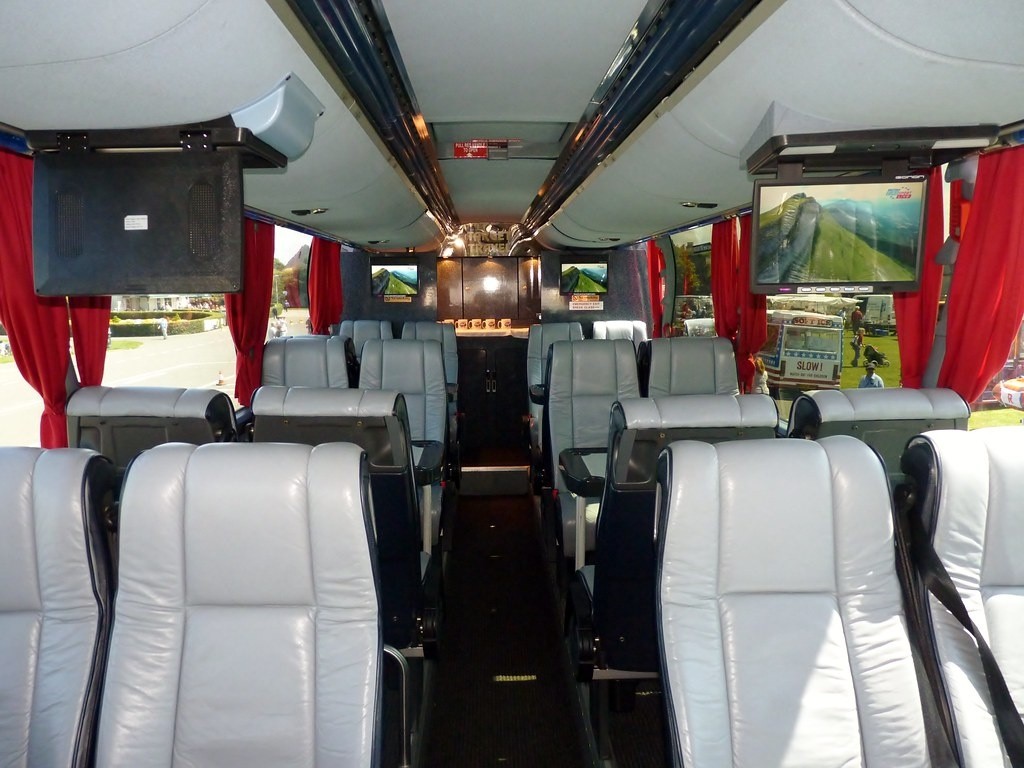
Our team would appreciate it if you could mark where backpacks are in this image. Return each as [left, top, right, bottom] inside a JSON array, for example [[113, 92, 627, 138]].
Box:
[[849, 334, 859, 349]]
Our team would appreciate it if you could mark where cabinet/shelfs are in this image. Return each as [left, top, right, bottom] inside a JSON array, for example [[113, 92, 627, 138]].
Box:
[[455, 336, 530, 470]]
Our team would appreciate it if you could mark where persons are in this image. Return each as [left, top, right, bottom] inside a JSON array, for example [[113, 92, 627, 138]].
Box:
[[157, 314, 168, 340], [284, 299, 289, 311], [269, 308, 286, 338], [837, 306, 879, 366], [858, 364, 884, 389]]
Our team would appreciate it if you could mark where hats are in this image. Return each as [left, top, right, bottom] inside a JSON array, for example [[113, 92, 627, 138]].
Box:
[[855, 306, 860, 309], [866, 363, 875, 370]]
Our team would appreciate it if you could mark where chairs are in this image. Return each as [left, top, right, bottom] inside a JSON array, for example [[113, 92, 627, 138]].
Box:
[[60, 384, 253, 597], [339, 321, 393, 364], [0, 446, 112, 768], [787, 386, 973, 513], [96, 441, 412, 768], [563, 393, 783, 768], [591, 320, 650, 364], [525, 321, 586, 453], [895, 429, 1024, 768], [637, 337, 740, 395], [356, 338, 453, 608], [648, 429, 955, 768], [250, 385, 443, 714], [261, 334, 357, 390], [528, 337, 645, 584], [400, 320, 460, 462]]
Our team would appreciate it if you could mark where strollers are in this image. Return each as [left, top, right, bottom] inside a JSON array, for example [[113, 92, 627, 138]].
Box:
[[862, 344, 891, 368]]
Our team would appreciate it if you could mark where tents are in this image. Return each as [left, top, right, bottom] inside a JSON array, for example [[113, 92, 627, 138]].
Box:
[[766, 294, 864, 312]]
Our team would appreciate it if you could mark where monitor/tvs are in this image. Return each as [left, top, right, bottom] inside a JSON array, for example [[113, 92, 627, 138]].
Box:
[[29, 152, 242, 296], [751, 178, 927, 293], [369, 257, 420, 297], [559, 261, 609, 295]]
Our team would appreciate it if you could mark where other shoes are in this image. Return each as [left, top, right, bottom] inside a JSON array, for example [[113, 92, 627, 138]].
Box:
[[851, 360, 858, 367]]
